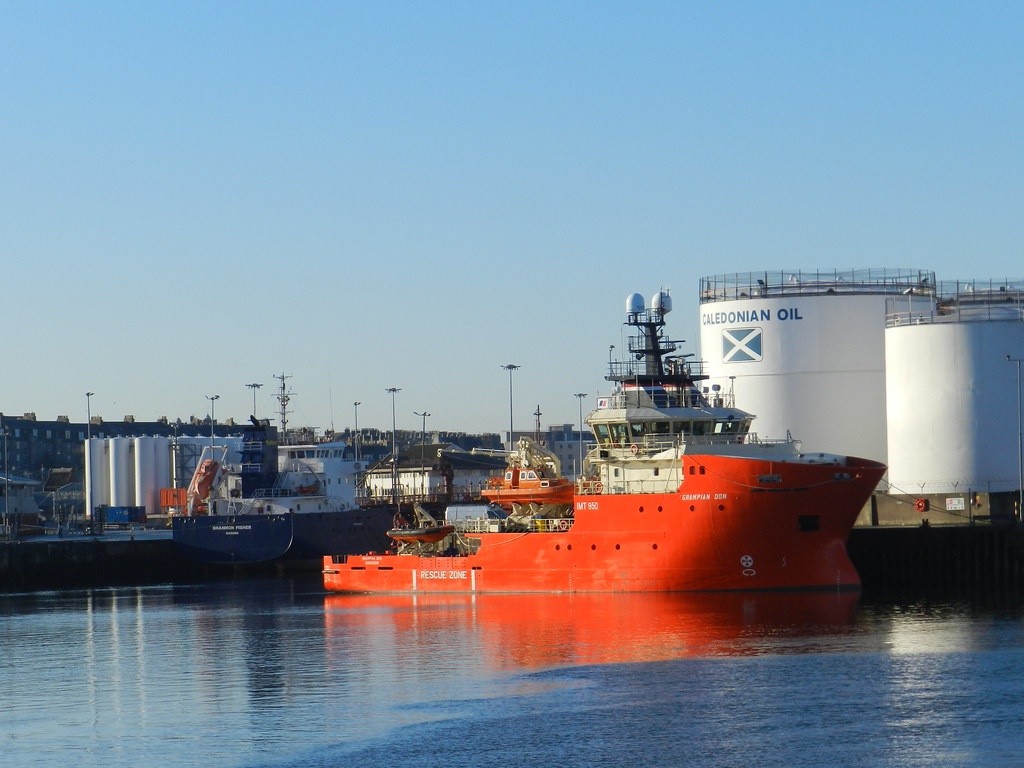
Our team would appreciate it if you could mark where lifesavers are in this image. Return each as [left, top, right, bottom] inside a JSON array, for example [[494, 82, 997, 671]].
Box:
[[630, 444, 638, 455], [914, 499, 926, 510], [595, 483, 603, 493], [559, 521, 568, 531]]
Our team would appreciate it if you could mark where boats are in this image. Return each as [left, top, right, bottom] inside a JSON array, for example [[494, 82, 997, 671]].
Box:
[[480, 474, 574, 508], [387, 519, 454, 543]]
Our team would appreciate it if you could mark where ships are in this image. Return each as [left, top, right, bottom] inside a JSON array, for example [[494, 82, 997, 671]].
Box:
[[319, 286, 888, 595]]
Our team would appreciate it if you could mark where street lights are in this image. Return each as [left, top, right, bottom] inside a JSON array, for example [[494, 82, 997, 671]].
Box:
[[573, 393, 588, 477], [245, 383, 263, 418], [383, 388, 402, 499], [353, 401, 362, 498], [413, 412, 431, 508], [500, 364, 521, 452], [205, 395, 220, 461], [86, 391, 95, 524], [0, 432, 12, 531]]
